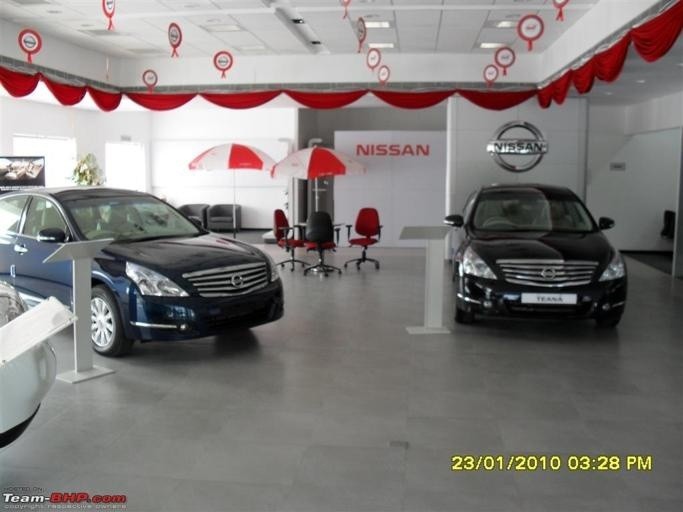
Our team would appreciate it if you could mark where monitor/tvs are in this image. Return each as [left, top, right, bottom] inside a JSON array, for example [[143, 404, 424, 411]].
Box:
[[0, 155, 46, 195]]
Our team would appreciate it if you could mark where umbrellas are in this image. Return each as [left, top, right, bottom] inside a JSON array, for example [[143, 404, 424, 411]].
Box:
[[271, 144, 367, 213], [189, 141, 278, 239]]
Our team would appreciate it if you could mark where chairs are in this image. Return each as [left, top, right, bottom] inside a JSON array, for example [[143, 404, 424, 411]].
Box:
[[274, 208, 380, 277], [176, 204, 241, 239]]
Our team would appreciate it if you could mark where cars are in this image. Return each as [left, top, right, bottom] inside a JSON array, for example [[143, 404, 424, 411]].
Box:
[[444, 183, 628, 327]]
